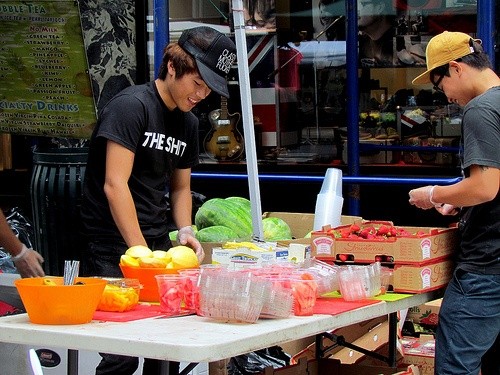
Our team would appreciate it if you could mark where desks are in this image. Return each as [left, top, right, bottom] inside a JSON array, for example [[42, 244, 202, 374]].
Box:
[[0, 287, 387, 375], [314, 286, 452, 375], [341, 138, 396, 163]]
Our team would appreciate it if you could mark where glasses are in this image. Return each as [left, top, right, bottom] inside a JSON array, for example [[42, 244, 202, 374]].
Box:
[[433, 61, 461, 92]]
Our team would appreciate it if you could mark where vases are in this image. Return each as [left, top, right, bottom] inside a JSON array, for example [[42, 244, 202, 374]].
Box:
[[370, 122, 391, 138]]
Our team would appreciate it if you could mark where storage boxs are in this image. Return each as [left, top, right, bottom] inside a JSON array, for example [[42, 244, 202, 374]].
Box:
[[197, 211, 458, 375]]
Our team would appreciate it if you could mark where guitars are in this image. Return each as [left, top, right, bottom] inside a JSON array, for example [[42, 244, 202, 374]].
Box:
[[203, 96, 244, 161]]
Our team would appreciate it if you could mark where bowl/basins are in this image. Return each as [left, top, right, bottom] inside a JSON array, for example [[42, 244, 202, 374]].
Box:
[[92, 277, 144, 312], [14, 277, 108, 325], [118, 263, 199, 302], [196, 258, 392, 323]]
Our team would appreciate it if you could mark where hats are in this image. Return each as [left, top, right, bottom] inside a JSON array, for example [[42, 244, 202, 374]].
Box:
[[413, 31, 482, 83], [178, 27, 236, 98]]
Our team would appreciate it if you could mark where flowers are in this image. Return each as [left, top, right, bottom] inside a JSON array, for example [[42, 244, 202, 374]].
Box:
[[360, 111, 395, 128]]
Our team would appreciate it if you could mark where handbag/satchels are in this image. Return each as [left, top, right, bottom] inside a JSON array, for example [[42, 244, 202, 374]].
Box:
[[225, 345, 291, 375]]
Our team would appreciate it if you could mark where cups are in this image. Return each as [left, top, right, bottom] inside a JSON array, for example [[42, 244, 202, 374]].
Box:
[[313, 167, 344, 231], [178, 269, 200, 309], [155, 274, 186, 314]]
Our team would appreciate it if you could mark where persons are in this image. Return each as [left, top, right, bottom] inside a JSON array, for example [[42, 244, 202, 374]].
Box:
[[0, 209, 45, 278], [318, 0, 346, 41], [408, 30, 500, 375], [77, 26, 237, 375], [357, 0, 396, 56]]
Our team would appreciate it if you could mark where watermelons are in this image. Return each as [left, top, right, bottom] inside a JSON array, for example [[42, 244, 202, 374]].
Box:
[[194, 196, 292, 242], [158, 266, 318, 315]]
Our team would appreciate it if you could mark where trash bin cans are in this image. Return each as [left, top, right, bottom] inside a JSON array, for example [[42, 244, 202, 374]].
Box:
[[30, 144, 90, 277]]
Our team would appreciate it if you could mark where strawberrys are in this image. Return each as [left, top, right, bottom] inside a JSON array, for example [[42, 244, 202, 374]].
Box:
[[327, 224, 426, 262]]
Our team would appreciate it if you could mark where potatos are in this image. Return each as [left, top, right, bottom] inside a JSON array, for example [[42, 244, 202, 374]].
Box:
[[120, 244, 199, 269]]
